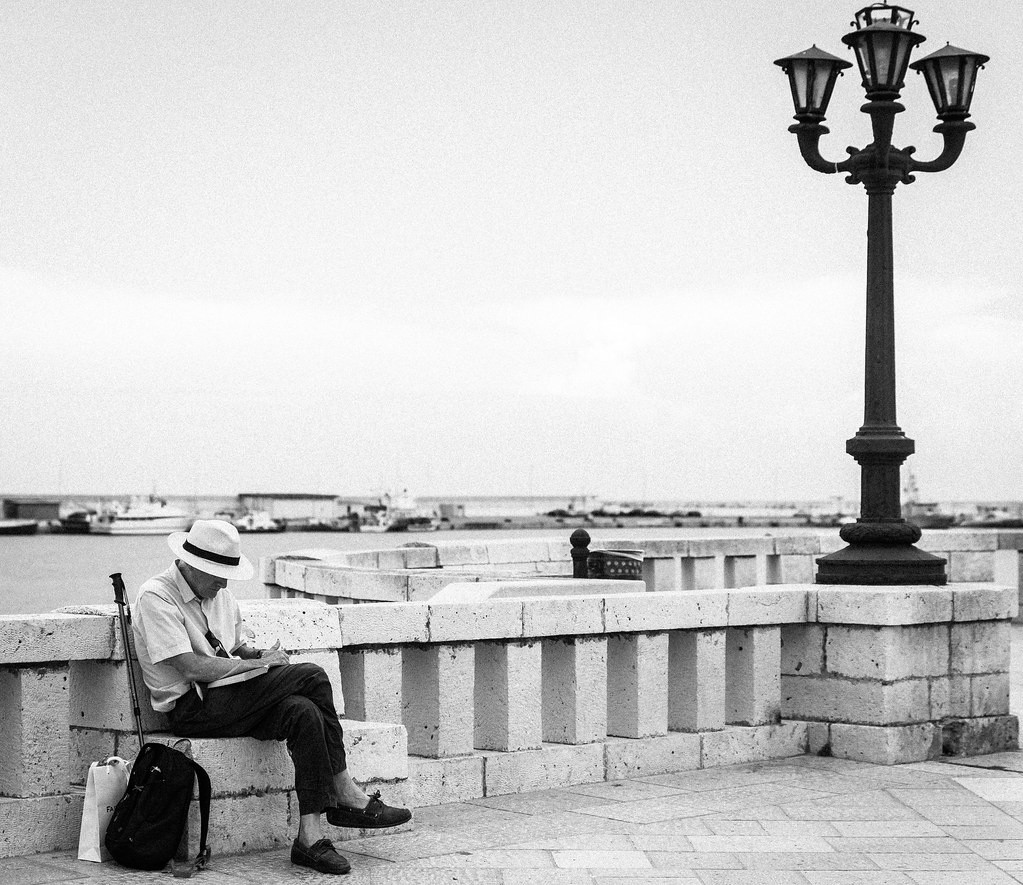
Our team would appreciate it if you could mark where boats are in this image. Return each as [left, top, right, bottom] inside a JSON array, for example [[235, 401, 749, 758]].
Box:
[[320, 493, 438, 533], [0, 515, 40, 537], [233, 508, 287, 533], [46, 520, 64, 536], [56, 504, 188, 535]]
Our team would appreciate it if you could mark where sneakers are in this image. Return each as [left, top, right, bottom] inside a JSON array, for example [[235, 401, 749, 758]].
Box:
[[290, 836, 351, 874], [326, 790, 412, 828]]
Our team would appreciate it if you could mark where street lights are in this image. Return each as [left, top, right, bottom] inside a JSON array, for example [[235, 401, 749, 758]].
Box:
[[769, 0, 989, 583]]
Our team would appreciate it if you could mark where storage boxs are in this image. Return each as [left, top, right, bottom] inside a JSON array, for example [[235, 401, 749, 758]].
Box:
[[587, 554, 645, 580]]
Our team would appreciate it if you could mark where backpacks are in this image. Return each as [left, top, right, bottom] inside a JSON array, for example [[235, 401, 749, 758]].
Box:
[[105, 738, 211, 878]]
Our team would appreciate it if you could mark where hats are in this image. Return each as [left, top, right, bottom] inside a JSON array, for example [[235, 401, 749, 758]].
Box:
[[168, 520, 255, 580]]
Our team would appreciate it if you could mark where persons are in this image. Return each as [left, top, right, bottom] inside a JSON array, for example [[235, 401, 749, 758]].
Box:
[[133, 520, 411, 875]]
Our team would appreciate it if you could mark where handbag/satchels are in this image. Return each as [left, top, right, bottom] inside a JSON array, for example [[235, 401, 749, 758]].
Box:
[[78, 756, 131, 863]]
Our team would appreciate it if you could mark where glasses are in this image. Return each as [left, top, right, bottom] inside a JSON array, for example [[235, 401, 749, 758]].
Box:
[[205, 630, 230, 659]]
